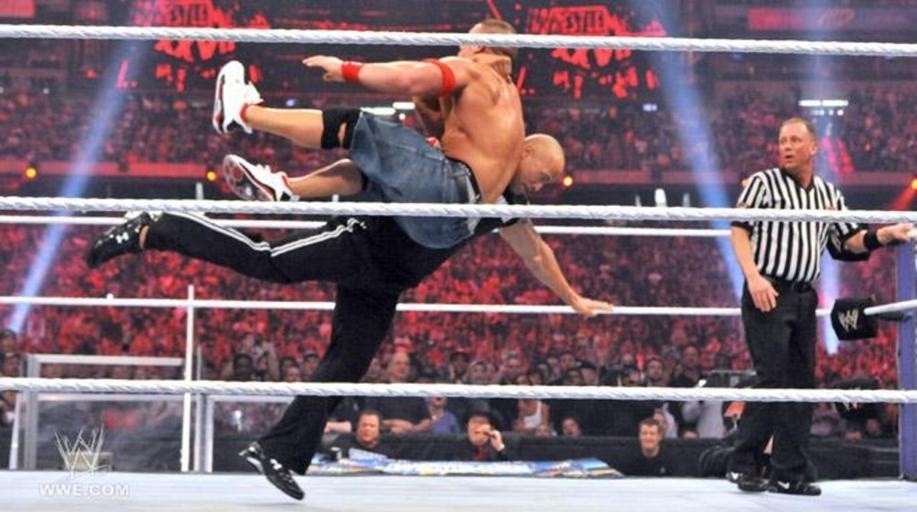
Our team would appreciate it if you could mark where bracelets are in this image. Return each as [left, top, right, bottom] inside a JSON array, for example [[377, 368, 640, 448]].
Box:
[[340, 60, 365, 87]]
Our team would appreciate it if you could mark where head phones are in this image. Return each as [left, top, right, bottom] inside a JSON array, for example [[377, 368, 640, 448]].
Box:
[[352, 409, 385, 433]]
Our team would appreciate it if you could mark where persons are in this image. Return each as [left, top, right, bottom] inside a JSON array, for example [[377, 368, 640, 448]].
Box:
[[732, 117, 915, 497], [212, 18, 529, 248], [77, 132, 615, 501], [0, 75, 916, 477]]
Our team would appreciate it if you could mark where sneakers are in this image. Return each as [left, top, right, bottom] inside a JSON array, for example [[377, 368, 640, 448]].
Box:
[[768, 477, 821, 496], [85, 210, 155, 270], [223, 153, 301, 202], [238, 442, 304, 500], [212, 59, 264, 135], [726, 470, 768, 491]]
